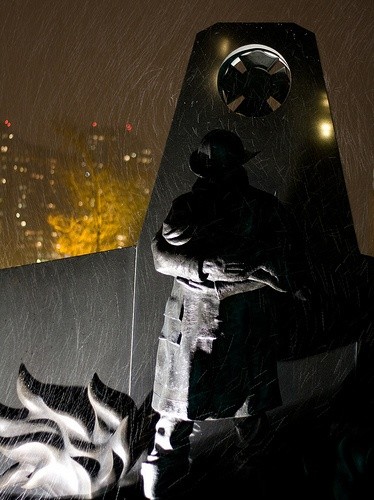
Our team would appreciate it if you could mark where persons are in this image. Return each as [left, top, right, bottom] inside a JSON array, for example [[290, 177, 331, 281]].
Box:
[[137, 128, 312, 500]]
